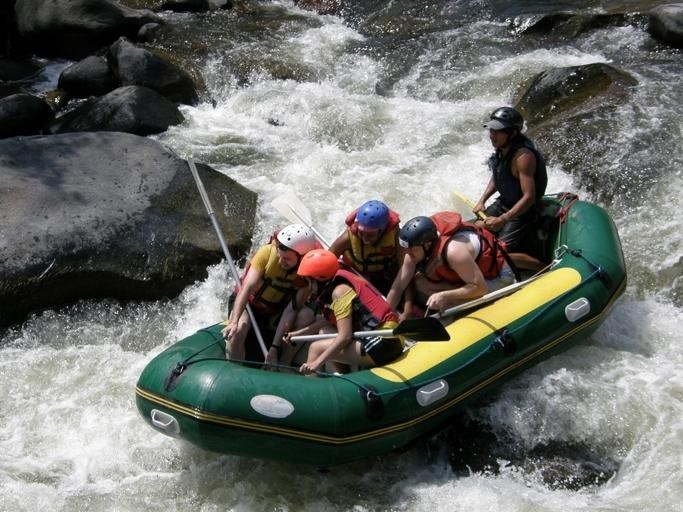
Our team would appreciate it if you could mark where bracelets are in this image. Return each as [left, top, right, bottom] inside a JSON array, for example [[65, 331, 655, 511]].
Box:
[[502, 213, 510, 222], [270, 345, 281, 351]]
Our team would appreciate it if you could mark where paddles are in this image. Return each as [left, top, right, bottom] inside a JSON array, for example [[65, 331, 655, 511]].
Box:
[[283, 318, 451, 348], [271, 190, 400, 314], [429, 274, 543, 320], [187, 156, 269, 362]]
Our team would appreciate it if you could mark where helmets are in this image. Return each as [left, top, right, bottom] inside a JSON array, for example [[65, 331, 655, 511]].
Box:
[[490, 107, 524, 132], [296, 249, 339, 281], [276, 224, 316, 255], [356, 200, 389, 236], [398, 215, 437, 249]]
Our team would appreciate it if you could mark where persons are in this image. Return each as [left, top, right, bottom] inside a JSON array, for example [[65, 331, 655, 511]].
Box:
[[472, 106, 547, 273], [220, 222, 315, 373], [281, 248, 406, 376], [385, 212, 516, 312], [328, 200, 414, 326]]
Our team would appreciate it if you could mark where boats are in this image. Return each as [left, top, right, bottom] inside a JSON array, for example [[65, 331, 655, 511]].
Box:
[[134, 192, 627, 467]]
